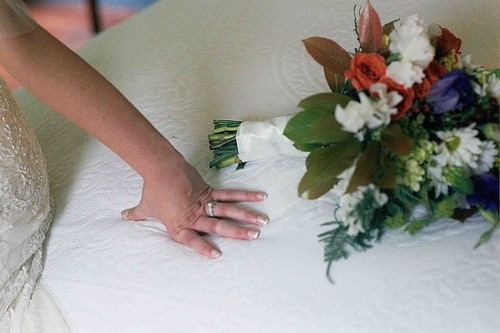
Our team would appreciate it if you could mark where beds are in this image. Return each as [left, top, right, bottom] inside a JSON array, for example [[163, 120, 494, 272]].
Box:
[[9, 0, 498, 333]]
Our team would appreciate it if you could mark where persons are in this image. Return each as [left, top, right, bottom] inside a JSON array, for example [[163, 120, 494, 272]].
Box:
[[0, 0, 270, 323]]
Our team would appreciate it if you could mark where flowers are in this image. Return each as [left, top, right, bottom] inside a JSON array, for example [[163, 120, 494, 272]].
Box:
[[208, 0, 499, 283]]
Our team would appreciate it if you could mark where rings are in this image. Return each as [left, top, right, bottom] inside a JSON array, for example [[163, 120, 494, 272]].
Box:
[[207, 200, 218, 218]]
